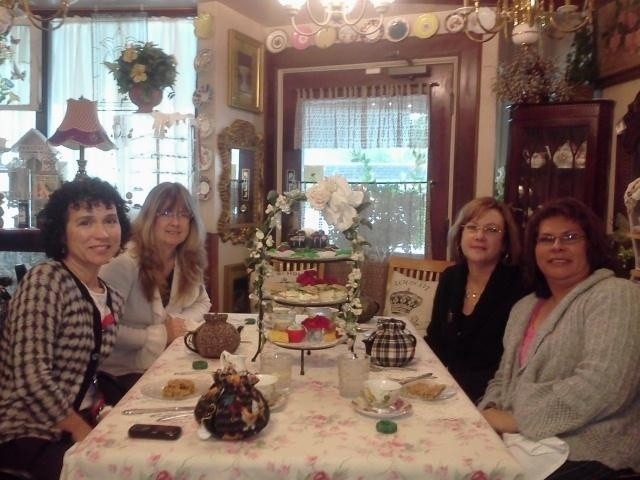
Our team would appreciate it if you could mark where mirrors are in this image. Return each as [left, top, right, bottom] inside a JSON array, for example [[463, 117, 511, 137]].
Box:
[[217, 119, 268, 245]]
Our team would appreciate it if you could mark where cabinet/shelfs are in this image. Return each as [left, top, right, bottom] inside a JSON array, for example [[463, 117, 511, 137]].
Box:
[[503, 99, 615, 234]]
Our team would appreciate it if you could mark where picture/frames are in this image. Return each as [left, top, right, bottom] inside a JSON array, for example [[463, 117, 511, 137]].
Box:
[[223, 262, 250, 313], [240, 168, 250, 201], [589, 0, 640, 89], [285, 169, 295, 193], [0, 15, 39, 111], [227, 29, 266, 113]]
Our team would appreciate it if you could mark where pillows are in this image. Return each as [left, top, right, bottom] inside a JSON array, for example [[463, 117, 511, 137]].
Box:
[[383, 271, 439, 337]]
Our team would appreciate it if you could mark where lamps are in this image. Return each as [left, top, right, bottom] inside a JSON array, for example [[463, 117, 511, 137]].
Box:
[[0, 0, 78, 31], [47, 96, 118, 179]]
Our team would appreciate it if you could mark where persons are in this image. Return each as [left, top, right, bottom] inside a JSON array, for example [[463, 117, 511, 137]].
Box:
[[1, 175, 129, 479], [474, 198, 639, 479], [421, 196, 538, 404], [97, 181, 211, 402]]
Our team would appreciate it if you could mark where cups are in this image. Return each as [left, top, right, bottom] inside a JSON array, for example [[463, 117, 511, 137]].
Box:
[[261, 353, 291, 392], [247, 374, 278, 405], [362, 381, 403, 408], [335, 352, 370, 398]]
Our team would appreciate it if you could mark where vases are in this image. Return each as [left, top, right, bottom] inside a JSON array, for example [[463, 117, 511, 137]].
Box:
[[129, 84, 162, 114]]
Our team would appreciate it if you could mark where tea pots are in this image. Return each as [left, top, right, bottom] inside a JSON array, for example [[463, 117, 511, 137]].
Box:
[[184, 312, 244, 358], [193, 364, 271, 441], [361, 318, 416, 367], [522, 140, 588, 170]]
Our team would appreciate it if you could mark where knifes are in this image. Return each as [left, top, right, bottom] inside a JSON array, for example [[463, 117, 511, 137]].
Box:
[[123, 406, 203, 415], [398, 372, 433, 385]]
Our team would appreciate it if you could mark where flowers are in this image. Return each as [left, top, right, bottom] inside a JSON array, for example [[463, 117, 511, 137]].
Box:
[[248, 172, 372, 349], [93, 34, 180, 99]]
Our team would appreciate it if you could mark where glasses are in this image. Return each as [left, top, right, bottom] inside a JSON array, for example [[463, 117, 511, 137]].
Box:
[[461, 223, 501, 235], [537, 231, 585, 244], [158, 209, 194, 220]]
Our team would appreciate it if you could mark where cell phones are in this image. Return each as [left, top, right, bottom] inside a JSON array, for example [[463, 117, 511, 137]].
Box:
[[128, 424, 181, 440]]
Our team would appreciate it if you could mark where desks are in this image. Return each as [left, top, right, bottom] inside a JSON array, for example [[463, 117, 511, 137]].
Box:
[[0, 227, 47, 252]]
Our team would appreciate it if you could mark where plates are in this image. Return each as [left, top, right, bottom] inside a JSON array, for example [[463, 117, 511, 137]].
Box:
[[413, 13, 439, 39], [265, 28, 288, 54], [192, 175, 213, 201], [141, 382, 216, 401], [338, 24, 358, 45], [194, 48, 216, 74], [192, 147, 213, 171], [402, 387, 457, 401], [291, 27, 313, 50], [316, 27, 337, 49], [351, 395, 411, 417], [468, 6, 496, 34], [192, 84, 216, 112], [196, 113, 214, 138], [445, 12, 466, 34], [194, 26, 214, 39], [360, 20, 385, 44], [385, 18, 410, 43]]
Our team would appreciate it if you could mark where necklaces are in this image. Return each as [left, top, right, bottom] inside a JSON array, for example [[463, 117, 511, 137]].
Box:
[[468, 272, 484, 299]]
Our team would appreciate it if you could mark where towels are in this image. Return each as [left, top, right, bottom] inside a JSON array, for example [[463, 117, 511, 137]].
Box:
[[499, 430, 569, 480]]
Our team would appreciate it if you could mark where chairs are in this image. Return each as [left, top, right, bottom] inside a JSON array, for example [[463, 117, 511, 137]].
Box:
[[15, 264, 29, 282], [385, 256, 451, 300]]
